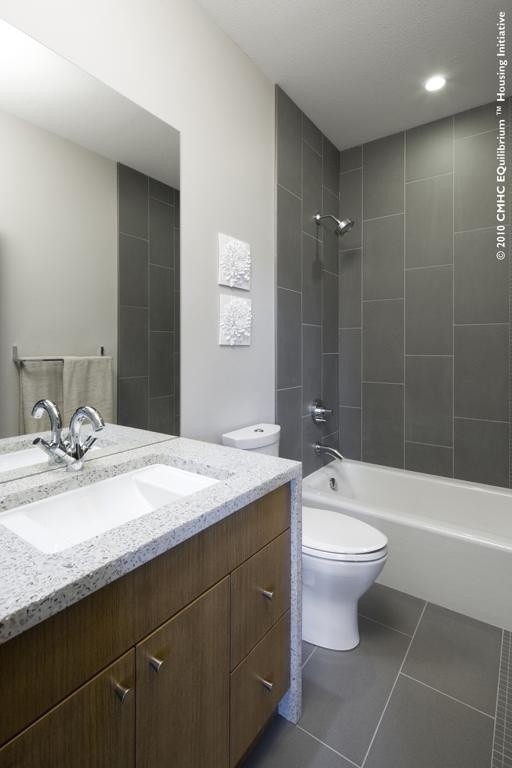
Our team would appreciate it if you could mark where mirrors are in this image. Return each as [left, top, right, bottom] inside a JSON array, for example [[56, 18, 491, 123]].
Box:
[[0, 18, 182, 488]]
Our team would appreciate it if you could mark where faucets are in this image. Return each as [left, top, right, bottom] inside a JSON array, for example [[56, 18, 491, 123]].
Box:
[[51, 406, 105, 472], [30, 396, 69, 461], [320, 444, 347, 464]]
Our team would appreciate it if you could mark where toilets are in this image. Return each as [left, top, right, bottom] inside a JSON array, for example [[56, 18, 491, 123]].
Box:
[[223, 423, 388, 651]]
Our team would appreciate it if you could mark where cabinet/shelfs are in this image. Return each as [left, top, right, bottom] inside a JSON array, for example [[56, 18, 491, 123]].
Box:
[[0, 478, 290, 768]]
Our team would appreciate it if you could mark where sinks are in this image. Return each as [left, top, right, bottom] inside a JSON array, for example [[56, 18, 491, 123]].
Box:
[[1, 455, 234, 555]]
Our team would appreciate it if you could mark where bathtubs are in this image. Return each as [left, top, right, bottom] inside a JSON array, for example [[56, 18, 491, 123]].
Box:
[[300, 460, 511, 635]]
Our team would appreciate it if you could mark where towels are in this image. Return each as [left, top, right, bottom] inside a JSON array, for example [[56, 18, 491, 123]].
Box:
[[17, 355, 113, 437]]
